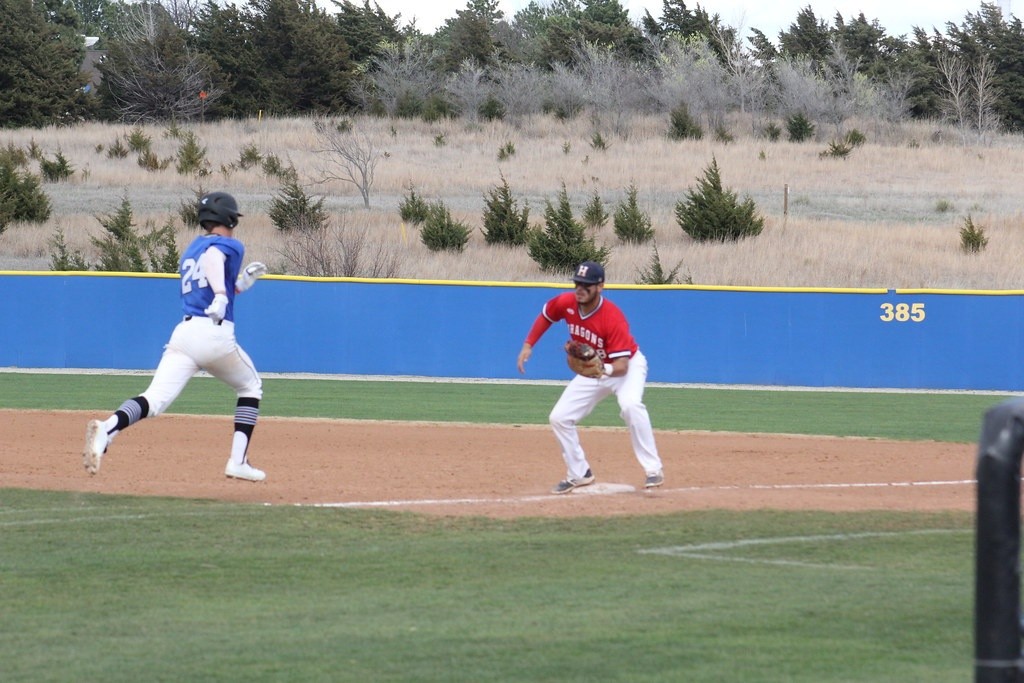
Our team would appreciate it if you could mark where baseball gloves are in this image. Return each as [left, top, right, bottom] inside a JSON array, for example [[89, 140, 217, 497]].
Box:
[[565, 339, 606, 379]]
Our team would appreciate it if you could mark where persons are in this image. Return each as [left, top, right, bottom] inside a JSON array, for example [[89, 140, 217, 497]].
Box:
[[517, 260, 665, 493], [82, 191, 266, 480]]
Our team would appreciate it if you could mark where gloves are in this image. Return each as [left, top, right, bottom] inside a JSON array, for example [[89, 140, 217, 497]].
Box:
[[235, 261, 268, 293], [204, 293, 230, 326]]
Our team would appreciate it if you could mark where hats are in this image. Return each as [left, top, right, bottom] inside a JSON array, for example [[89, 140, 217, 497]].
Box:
[[569, 262, 606, 285]]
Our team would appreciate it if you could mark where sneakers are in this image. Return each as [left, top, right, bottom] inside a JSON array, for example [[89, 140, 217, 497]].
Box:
[[81, 419, 110, 474], [224, 458, 266, 482], [645, 476, 663, 488], [549, 469, 596, 494]]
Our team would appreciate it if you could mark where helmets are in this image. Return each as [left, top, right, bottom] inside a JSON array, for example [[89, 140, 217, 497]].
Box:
[[197, 192, 243, 229]]
